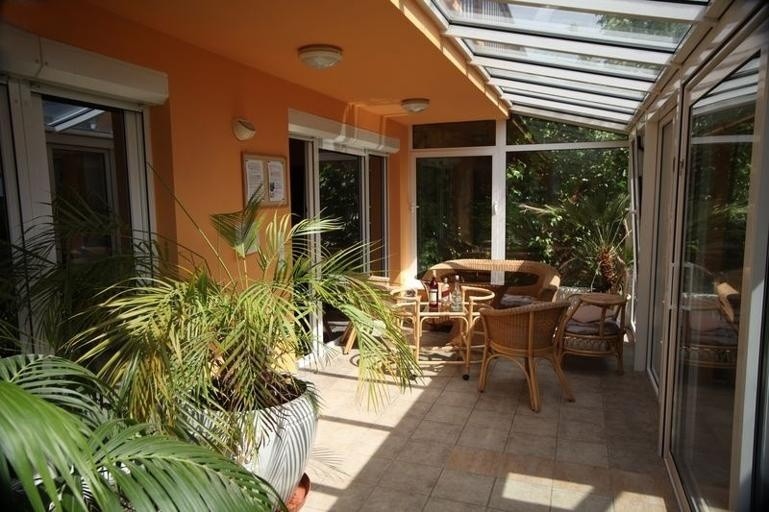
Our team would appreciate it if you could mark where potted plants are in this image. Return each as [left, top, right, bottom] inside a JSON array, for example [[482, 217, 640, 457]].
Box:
[[0, 177, 423, 511]]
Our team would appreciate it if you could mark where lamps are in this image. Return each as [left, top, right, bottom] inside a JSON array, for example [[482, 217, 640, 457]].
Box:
[[400, 98, 430, 113], [299, 44, 343, 70]]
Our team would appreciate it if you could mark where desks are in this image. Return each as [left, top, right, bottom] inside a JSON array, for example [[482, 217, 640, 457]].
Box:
[[557, 289, 627, 378]]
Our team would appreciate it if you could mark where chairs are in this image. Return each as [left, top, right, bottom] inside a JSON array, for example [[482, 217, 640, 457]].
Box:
[[338, 284, 576, 414]]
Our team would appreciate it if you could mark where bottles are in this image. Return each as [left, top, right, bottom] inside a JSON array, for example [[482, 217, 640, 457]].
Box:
[[429, 275, 463, 312]]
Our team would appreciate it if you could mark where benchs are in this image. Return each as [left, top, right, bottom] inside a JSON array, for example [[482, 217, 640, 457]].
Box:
[[422, 257, 561, 314]]
